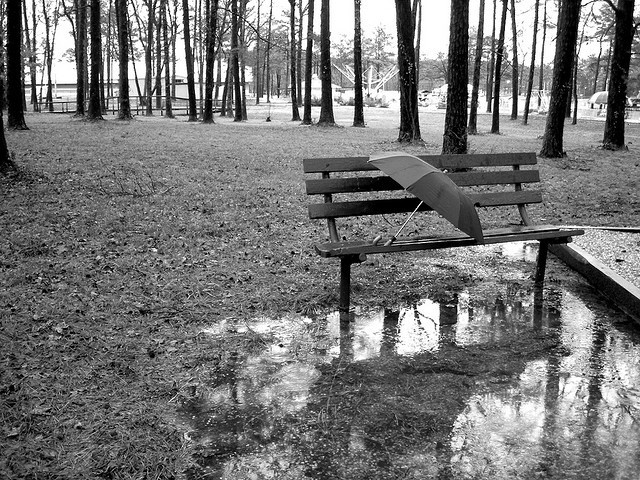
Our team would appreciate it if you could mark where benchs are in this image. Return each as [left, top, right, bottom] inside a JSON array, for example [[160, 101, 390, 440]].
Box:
[[303, 151, 585, 315]]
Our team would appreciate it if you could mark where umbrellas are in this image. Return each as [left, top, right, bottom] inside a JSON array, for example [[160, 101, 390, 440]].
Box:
[[367, 151, 487, 245], [587, 91, 633, 107]]
